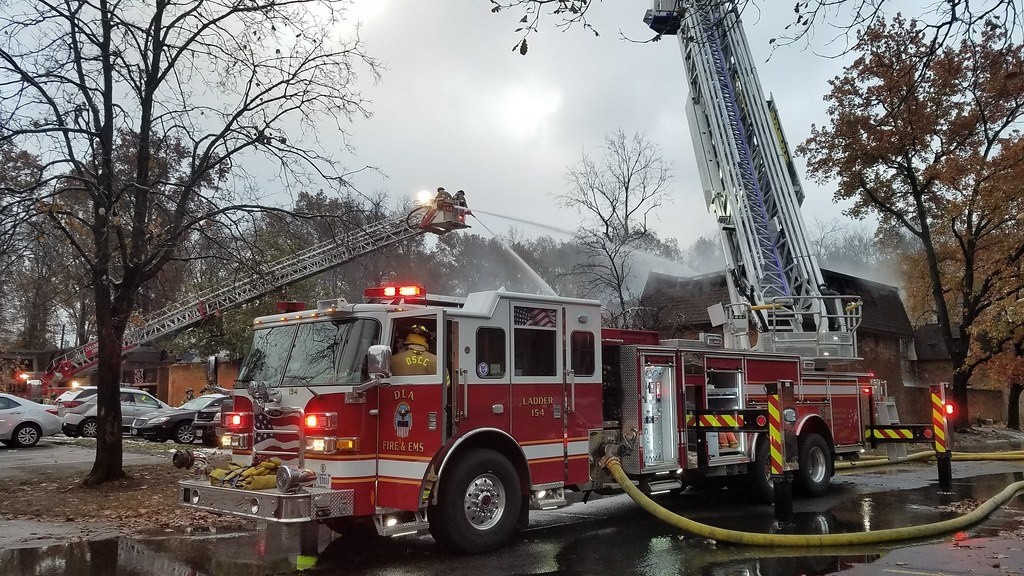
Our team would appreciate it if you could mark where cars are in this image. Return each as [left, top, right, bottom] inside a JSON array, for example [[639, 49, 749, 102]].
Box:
[[52, 385, 100, 417], [128, 392, 230, 445], [191, 396, 231, 445], [62, 388, 179, 438], [0, 392, 63, 449]]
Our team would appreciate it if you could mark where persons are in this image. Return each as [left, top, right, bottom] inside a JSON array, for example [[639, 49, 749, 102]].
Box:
[[141, 388, 151, 401], [47, 392, 58, 405], [181, 387, 197, 406], [390, 325, 450, 387], [431, 186, 452, 208], [453, 189, 468, 208], [160, 347, 177, 360], [392, 335, 406, 355]]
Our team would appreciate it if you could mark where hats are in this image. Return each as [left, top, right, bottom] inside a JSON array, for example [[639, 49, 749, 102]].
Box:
[[458, 190, 465, 195], [437, 187, 445, 192]]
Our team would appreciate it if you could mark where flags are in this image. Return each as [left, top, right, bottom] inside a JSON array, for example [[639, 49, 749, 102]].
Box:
[[514, 306, 556, 327]]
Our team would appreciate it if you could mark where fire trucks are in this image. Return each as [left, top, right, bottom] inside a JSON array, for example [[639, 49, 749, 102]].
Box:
[[170, 0, 958, 555], [8, 199, 471, 406]]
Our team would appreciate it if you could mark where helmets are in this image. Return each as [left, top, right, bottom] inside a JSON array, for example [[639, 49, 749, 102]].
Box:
[[402, 333, 429, 351]]
